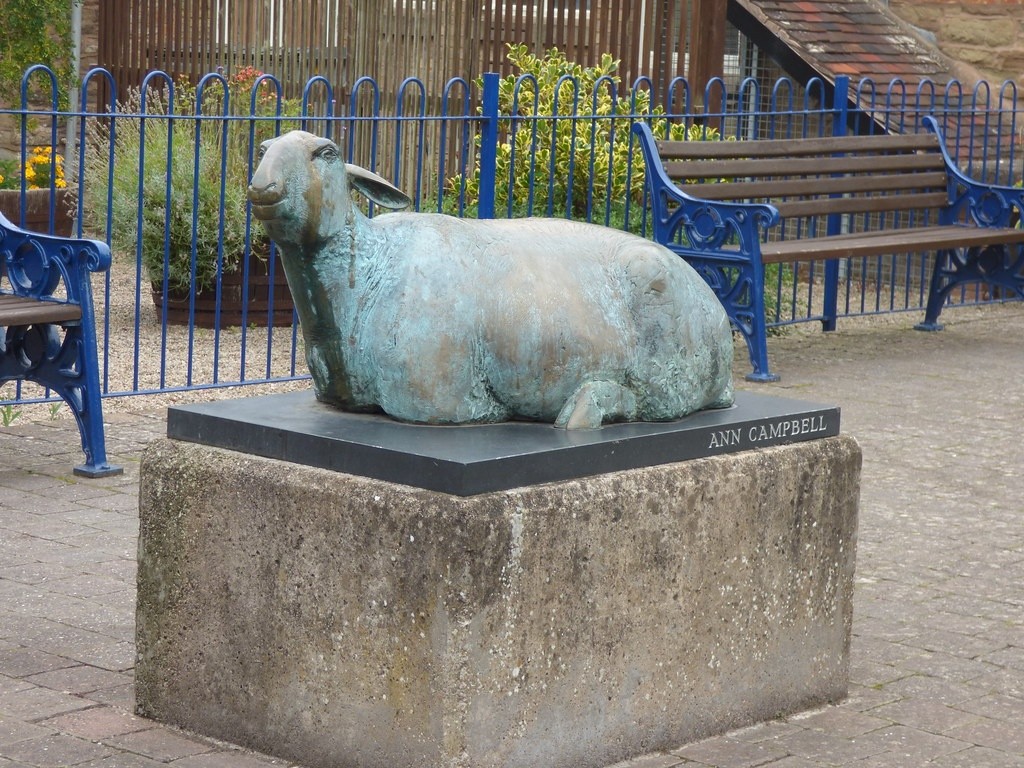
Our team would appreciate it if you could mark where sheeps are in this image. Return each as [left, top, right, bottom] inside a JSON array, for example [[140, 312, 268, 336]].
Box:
[[247, 130, 734, 431]]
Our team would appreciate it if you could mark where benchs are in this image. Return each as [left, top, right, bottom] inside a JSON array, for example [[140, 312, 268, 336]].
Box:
[[0, 211, 125, 480], [631, 112, 1024, 386]]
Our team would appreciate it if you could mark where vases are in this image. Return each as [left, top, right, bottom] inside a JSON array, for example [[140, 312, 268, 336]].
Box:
[[0, 181, 79, 276]]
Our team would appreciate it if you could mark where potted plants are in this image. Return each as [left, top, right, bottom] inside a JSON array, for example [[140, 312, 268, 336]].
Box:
[[70, 66, 313, 327]]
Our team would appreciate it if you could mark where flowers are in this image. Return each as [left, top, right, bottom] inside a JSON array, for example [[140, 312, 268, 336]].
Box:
[[0, 146, 68, 190]]
